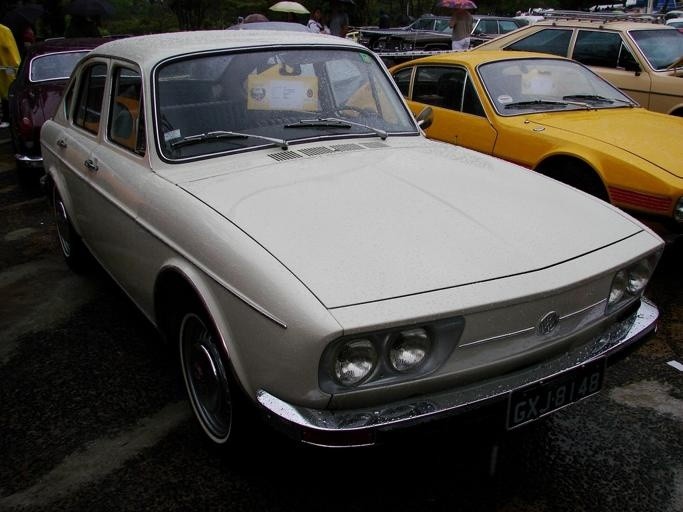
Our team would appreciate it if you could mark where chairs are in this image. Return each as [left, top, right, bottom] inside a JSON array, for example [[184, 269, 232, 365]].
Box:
[[92, 79, 312, 148], [393, 72, 543, 116]]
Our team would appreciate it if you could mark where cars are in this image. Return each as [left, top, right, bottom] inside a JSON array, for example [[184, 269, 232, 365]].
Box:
[[8, 36, 146, 171], [346, 5, 683, 67], [40, 28, 667, 460], [470, 18, 683, 118], [343, 49, 683, 223]]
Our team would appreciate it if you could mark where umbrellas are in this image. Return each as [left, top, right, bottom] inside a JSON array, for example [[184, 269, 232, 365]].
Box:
[[268, 0, 312, 22], [435, 0, 478, 19]]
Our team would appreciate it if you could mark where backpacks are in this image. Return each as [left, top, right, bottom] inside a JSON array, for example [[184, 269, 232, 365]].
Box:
[[321, 24, 331, 34]]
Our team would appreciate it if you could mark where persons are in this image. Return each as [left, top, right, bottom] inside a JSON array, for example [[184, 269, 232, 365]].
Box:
[[448, 8, 473, 84], [16, 26, 35, 61], [308, 7, 328, 35], [241, 7, 269, 23], [328, 1, 350, 38], [283, 13, 298, 23], [378, 8, 389, 45]]
